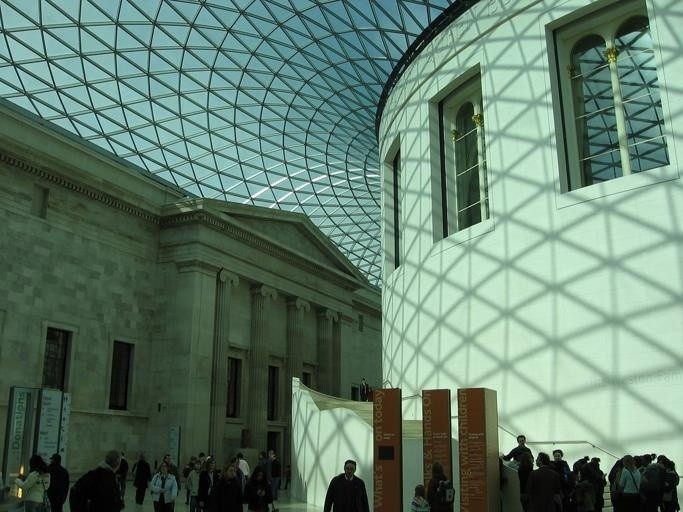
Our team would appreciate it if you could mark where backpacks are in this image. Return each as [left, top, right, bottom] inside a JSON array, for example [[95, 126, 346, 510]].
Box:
[[70, 472, 98, 511]]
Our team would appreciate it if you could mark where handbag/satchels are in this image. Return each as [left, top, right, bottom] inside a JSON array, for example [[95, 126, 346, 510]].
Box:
[[43, 489, 52, 510]]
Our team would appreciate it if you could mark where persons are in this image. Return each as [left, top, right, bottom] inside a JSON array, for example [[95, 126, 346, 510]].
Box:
[[410, 484, 430, 512], [499, 434, 680, 512], [15, 449, 281, 511], [323, 460, 369, 512], [425, 462, 455, 512], [360, 377, 369, 402]]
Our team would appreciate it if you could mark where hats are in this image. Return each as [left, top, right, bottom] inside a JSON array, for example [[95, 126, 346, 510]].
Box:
[[49, 454, 61, 461]]
